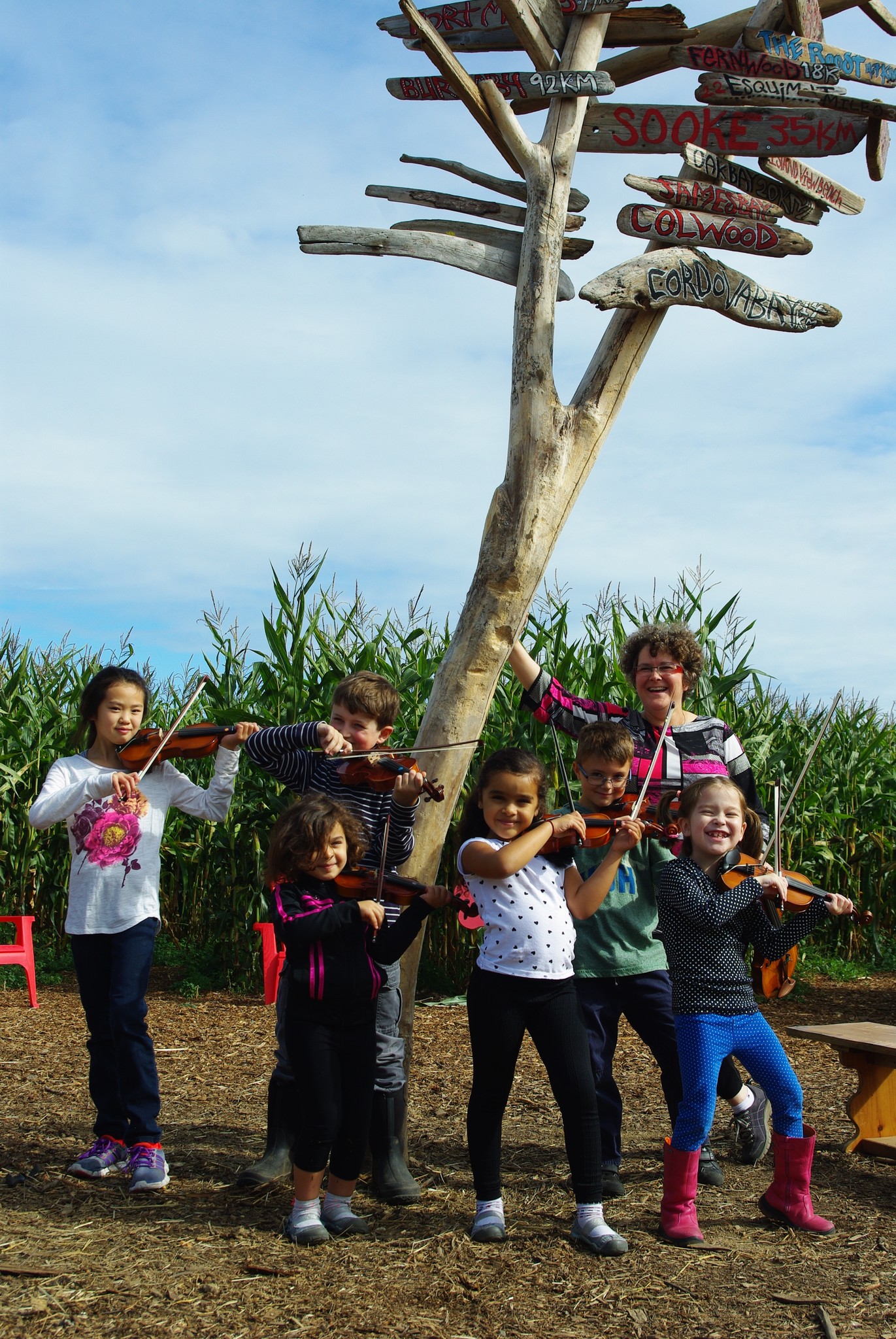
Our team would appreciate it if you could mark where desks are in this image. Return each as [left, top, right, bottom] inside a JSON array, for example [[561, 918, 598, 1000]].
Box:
[[783, 1018, 896, 1155]]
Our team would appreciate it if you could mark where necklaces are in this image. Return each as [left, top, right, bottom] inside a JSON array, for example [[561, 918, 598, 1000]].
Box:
[[683, 710, 685, 724]]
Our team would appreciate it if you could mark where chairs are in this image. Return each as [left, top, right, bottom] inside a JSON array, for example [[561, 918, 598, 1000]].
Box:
[[0, 914, 43, 1008], [252, 921, 286, 1005]]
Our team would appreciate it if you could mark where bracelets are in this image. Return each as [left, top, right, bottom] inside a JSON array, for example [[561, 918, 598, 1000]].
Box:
[[545, 820, 554, 838]]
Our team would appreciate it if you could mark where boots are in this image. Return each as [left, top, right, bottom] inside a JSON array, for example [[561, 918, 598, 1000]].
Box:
[[758, 1123, 836, 1235], [658, 1137, 704, 1247], [370, 1088, 421, 1206], [237, 1078, 294, 1188]]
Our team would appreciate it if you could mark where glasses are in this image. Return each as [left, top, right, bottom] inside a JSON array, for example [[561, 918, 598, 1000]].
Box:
[[634, 663, 684, 677], [577, 762, 632, 788]]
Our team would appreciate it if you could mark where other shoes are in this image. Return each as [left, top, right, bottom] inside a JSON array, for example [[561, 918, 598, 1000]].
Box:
[[320, 1208, 370, 1235], [570, 1217, 629, 1257], [601, 1164, 625, 1196], [287, 1212, 329, 1246], [471, 1210, 506, 1243]]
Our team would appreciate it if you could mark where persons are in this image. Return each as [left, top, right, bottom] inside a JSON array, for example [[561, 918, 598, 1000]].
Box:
[[549, 720, 725, 1196], [507, 613, 773, 1166], [238, 670, 427, 1206], [453, 747, 645, 1255], [258, 792, 453, 1245], [29, 665, 260, 1192], [656, 776, 853, 1247]]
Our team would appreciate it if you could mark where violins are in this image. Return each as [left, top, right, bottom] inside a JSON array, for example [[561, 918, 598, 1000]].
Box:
[[512, 812, 679, 853], [334, 862, 478, 919], [603, 793, 682, 834], [114, 720, 269, 773], [722, 851, 873, 923], [336, 746, 445, 802], [749, 892, 798, 999]]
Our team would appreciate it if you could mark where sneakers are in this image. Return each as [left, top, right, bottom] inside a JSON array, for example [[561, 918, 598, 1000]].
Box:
[[68, 1135, 133, 1180], [120, 1143, 170, 1194], [697, 1135, 725, 1186], [728, 1077, 772, 1165]]
